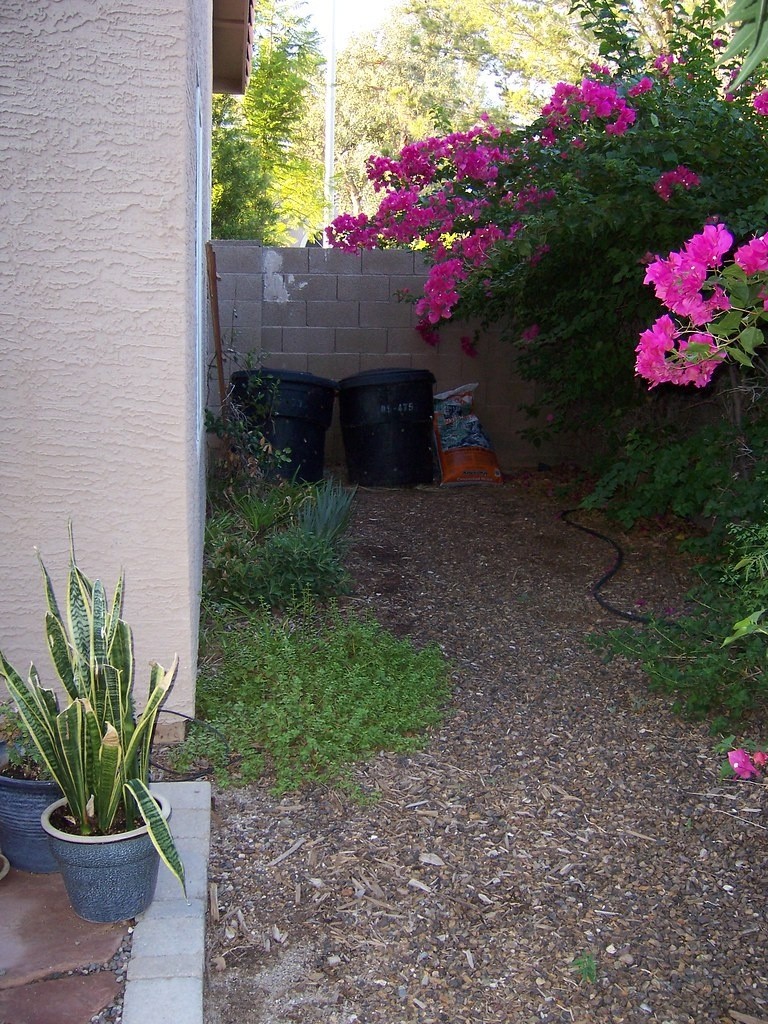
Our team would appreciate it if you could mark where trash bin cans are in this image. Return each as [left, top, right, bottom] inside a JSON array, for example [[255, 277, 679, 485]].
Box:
[[337, 367, 436, 489], [227, 370, 335, 492]]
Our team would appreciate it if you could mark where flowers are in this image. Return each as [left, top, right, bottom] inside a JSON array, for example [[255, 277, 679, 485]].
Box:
[[0, 698, 58, 782]]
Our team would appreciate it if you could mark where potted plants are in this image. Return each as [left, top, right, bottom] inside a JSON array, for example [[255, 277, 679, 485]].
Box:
[[0, 517, 192, 925]]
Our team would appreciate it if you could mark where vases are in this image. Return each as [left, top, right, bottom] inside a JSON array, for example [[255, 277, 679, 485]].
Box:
[[0, 755, 66, 874], [0, 722, 24, 767]]
